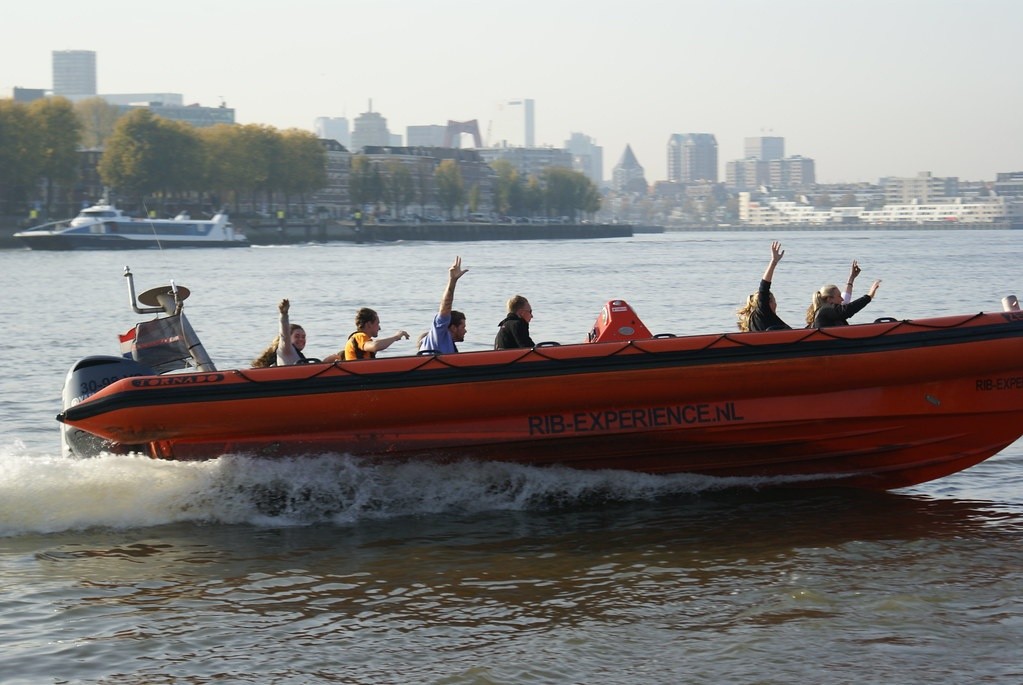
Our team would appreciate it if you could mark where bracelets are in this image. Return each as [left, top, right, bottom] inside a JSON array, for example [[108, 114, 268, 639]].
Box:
[[846, 283, 853, 287]]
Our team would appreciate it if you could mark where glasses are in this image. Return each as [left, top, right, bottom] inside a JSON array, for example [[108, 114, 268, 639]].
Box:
[[522, 309, 532, 314]]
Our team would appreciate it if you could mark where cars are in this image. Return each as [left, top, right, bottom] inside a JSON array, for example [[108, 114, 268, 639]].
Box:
[[467, 212, 569, 224]]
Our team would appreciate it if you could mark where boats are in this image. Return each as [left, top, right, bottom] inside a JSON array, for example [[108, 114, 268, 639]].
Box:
[[55, 295, 1022, 492], [12, 185, 251, 251]]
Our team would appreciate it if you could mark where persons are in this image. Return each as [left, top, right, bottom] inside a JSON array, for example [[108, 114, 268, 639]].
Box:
[[813, 279, 880, 327], [806, 261, 861, 323], [416, 255, 466, 354], [251, 299, 341, 366], [342, 308, 409, 360], [737, 242, 792, 332], [493, 295, 534, 349]]
